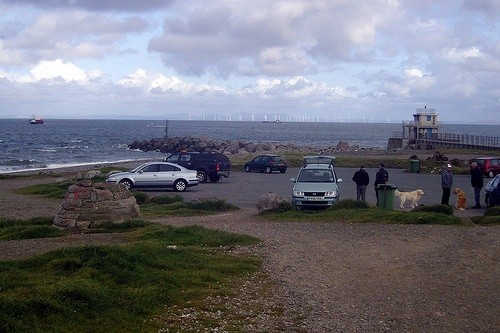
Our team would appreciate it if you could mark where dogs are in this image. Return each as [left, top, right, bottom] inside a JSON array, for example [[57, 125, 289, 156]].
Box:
[[452, 188, 467, 211], [394, 189, 425, 209]]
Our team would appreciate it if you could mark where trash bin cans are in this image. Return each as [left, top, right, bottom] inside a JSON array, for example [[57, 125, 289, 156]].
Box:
[[408, 158, 421, 175], [375, 183, 397, 211]]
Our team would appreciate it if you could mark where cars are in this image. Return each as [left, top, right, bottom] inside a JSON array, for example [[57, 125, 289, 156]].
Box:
[[484, 174, 500, 208], [105, 162, 200, 192], [244, 154, 288, 174]]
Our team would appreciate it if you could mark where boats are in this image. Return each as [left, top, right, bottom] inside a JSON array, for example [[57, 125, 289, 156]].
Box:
[[263, 119, 282, 123], [30, 113, 44, 124]]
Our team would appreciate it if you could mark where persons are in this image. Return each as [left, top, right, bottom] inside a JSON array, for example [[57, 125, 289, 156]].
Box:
[[470, 161, 483, 209], [374, 164, 389, 206], [352, 166, 369, 200], [441, 163, 453, 206]]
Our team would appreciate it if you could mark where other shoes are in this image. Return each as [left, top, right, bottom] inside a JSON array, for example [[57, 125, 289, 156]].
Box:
[[471, 206, 481, 209]]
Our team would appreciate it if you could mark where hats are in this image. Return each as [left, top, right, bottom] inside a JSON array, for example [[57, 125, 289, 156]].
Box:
[[446, 164, 452, 167], [361, 164, 367, 168]]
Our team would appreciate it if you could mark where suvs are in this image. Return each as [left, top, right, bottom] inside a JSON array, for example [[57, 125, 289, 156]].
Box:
[[160, 150, 231, 183], [471, 157, 500, 178], [289, 155, 343, 210]]
